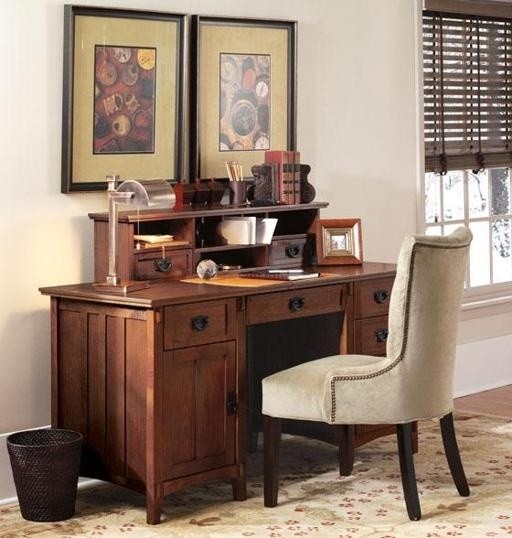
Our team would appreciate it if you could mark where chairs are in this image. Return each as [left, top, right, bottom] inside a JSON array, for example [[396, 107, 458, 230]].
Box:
[[257, 224, 479, 523]]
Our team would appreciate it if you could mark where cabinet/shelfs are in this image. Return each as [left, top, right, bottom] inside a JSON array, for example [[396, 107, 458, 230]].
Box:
[[78, 202, 332, 283]]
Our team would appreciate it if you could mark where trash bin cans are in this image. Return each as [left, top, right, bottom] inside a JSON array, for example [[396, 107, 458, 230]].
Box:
[[6, 429, 84, 522]]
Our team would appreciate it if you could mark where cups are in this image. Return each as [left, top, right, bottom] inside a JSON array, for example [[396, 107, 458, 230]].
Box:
[[228, 181, 247, 208]]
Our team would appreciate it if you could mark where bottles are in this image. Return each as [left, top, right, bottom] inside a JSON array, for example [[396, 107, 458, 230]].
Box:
[[299, 164, 315, 203], [174, 176, 225, 212]]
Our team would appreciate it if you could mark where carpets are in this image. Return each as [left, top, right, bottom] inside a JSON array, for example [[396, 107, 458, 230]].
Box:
[[1, 406, 512, 538]]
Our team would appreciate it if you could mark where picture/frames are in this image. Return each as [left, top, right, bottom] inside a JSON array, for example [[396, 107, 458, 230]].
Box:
[[56, 1, 193, 195], [315, 216, 364, 268], [190, 12, 304, 180]]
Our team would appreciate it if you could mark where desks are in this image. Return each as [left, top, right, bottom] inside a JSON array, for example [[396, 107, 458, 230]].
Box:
[[38, 256, 423, 526]]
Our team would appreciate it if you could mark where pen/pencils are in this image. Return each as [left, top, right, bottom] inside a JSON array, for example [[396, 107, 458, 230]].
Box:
[[226, 161, 243, 181], [268, 269, 305, 274]]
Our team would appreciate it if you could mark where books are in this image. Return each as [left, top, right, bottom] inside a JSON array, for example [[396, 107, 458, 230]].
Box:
[[239, 267, 321, 281], [264, 151, 302, 206]]
